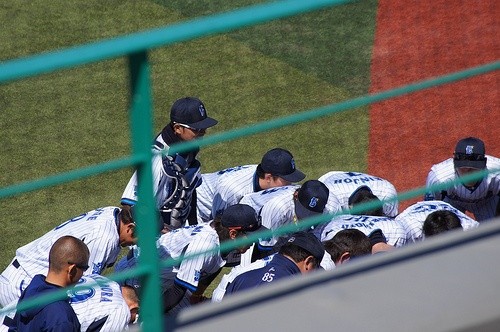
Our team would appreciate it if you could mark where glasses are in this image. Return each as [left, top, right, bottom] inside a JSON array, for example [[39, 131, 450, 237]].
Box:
[[68, 261, 89, 272], [174, 123, 206, 134]]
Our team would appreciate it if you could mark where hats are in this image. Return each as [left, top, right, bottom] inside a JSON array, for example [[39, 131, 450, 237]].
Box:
[[130, 203, 165, 231], [262, 147, 306, 182], [127, 284, 142, 300], [452, 137, 487, 171], [222, 204, 272, 235], [169, 96, 218, 130], [287, 230, 324, 270], [295, 179, 329, 220]]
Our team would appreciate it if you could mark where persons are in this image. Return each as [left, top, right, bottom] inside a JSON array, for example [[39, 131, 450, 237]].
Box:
[[119, 96, 218, 230], [319, 230, 371, 269], [67, 273, 158, 332], [424, 137, 500, 222], [114, 204, 270, 305], [1, 237, 89, 332], [312, 216, 405, 256], [240, 179, 329, 234], [317, 170, 397, 213], [0, 203, 138, 323], [395, 200, 478, 247], [198, 147, 305, 216], [224, 230, 324, 301]]
